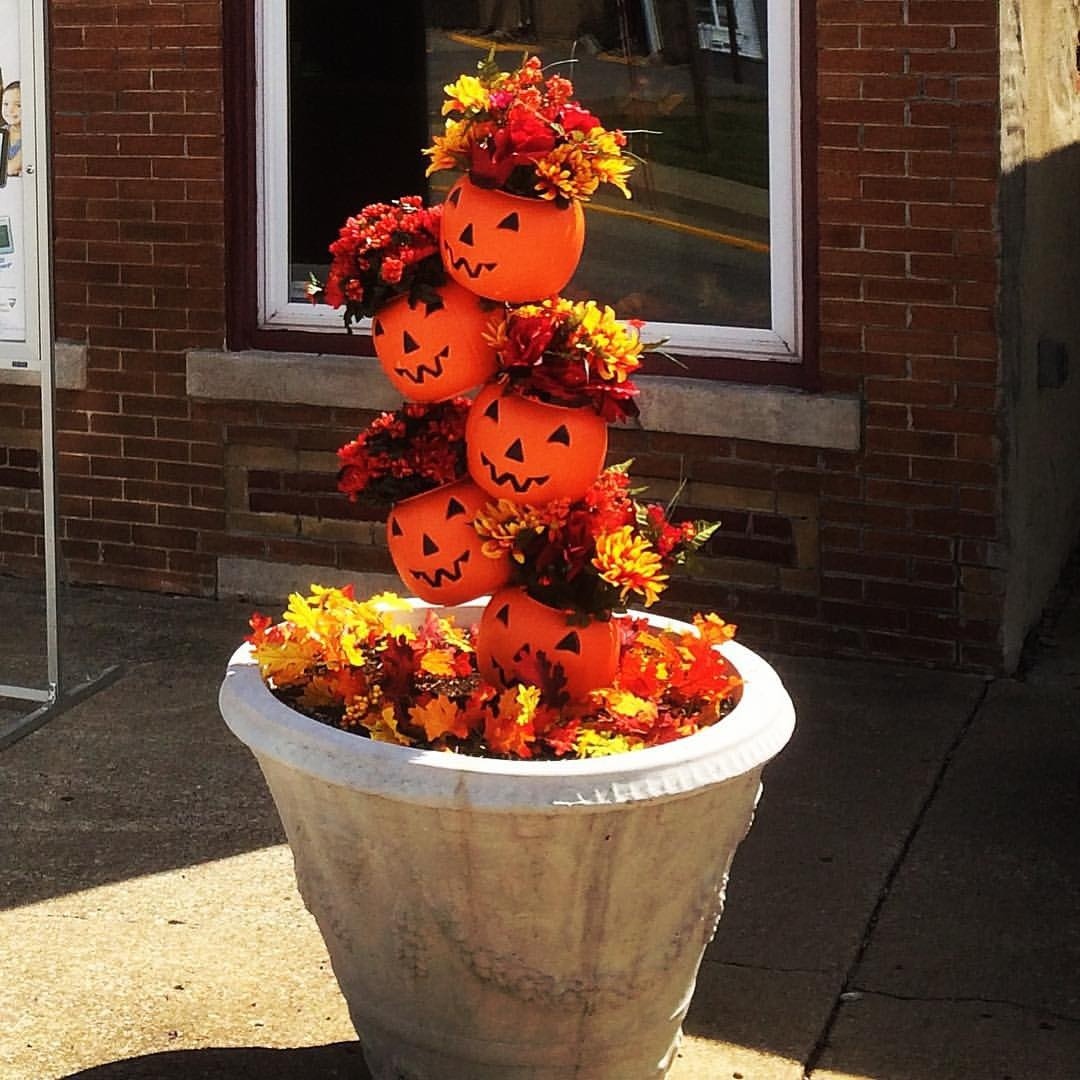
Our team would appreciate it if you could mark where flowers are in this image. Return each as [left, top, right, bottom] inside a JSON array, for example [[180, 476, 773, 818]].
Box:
[[240, 43, 741, 759]]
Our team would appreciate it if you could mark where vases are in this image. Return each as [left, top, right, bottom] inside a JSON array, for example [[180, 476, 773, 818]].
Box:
[[218, 596, 795, 1080]]
